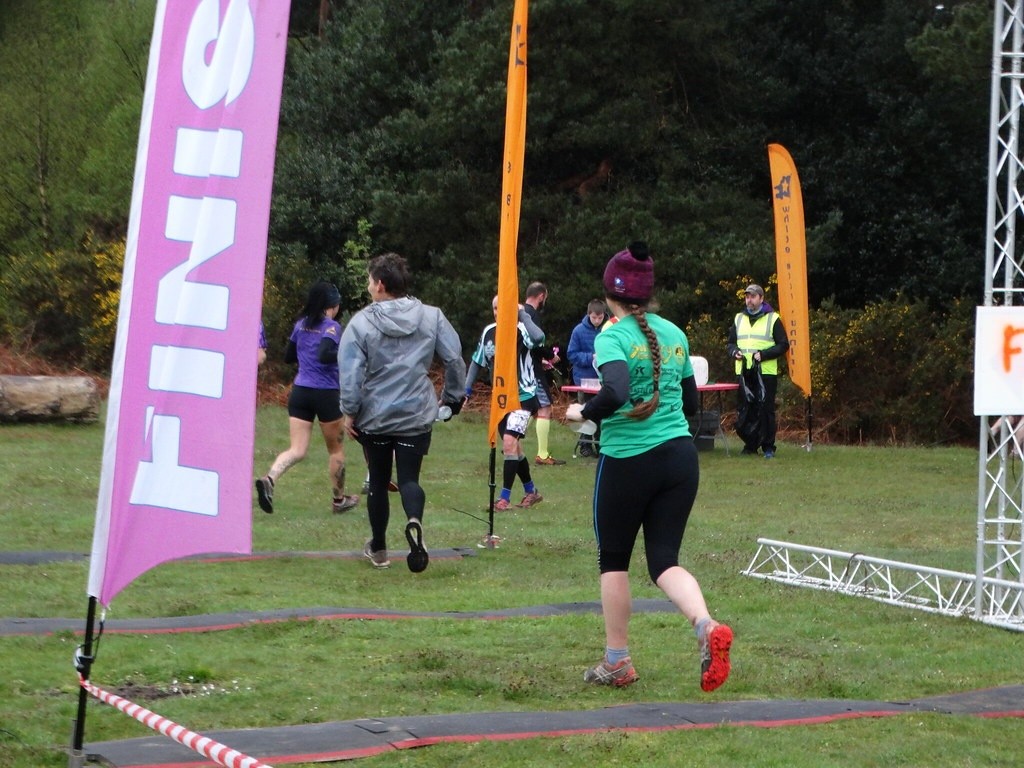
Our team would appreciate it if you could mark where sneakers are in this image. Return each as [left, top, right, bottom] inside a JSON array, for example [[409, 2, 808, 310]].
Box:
[[741, 447, 758, 455], [486, 497, 511, 512], [364, 537, 392, 569], [405, 517, 429, 572], [332, 495, 359, 513], [698, 619, 733, 692], [584, 652, 640, 690], [515, 492, 543, 509], [535, 455, 566, 465], [764, 447, 775, 460], [256, 476, 274, 513]]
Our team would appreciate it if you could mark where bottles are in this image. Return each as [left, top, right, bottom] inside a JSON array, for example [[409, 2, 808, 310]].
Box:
[[435, 406, 451, 422]]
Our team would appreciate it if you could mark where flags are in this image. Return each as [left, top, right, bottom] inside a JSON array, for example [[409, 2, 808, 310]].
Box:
[[100, 0, 290, 599], [489, 1, 528, 447], [766, 145, 811, 395]]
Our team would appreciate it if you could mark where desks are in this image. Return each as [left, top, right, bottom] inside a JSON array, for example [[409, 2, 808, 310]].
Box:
[[559, 383, 739, 461]]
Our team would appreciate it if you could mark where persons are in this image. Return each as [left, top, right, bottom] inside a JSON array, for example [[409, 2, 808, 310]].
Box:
[[468, 291, 544, 511], [729, 283, 789, 461], [525, 281, 567, 467], [338, 252, 466, 574], [256, 280, 360, 515], [562, 237, 746, 692]]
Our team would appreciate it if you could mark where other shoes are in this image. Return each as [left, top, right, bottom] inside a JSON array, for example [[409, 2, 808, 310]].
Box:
[[580, 434, 594, 456]]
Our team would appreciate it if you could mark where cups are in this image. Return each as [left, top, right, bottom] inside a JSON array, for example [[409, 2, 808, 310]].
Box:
[[581, 379, 601, 391], [569, 419, 597, 435]]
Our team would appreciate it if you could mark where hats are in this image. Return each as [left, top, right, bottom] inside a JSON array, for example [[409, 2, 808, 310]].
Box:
[[744, 284, 764, 296], [324, 284, 341, 307], [603, 241, 654, 306]]
